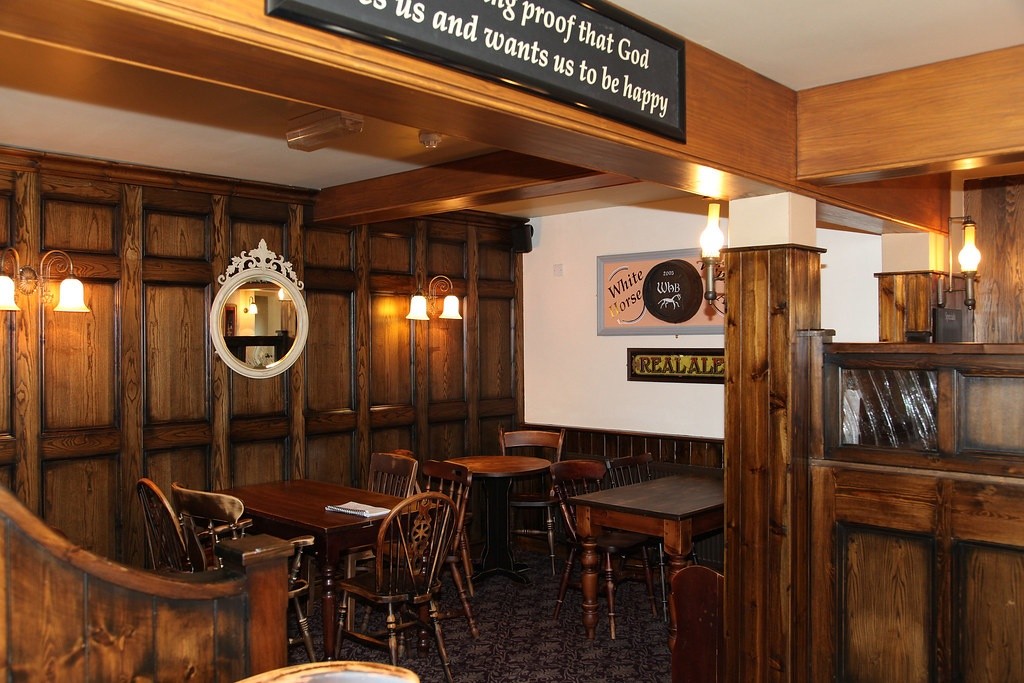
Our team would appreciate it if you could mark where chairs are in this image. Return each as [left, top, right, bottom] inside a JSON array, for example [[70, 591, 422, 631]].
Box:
[[552, 459, 657, 621], [136, 449, 482, 683], [606, 452, 699, 621], [497, 426, 569, 555]]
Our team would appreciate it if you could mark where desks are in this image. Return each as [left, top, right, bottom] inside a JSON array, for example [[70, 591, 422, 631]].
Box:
[[572, 475, 722, 639], [206, 479, 426, 658], [436, 455, 552, 586]]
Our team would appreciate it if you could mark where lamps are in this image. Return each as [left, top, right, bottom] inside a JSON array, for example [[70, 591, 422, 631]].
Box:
[[935, 216, 982, 310], [701, 199, 737, 310], [0, 247, 92, 311], [405, 269, 462, 321], [246, 296, 258, 314], [286, 108, 364, 150]]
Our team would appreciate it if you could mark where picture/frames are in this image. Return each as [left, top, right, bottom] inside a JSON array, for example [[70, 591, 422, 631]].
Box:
[[222, 303, 237, 337], [596, 245, 725, 336]]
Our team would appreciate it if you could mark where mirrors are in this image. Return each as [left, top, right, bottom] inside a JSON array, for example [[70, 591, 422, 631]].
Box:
[[209, 239, 312, 377]]
